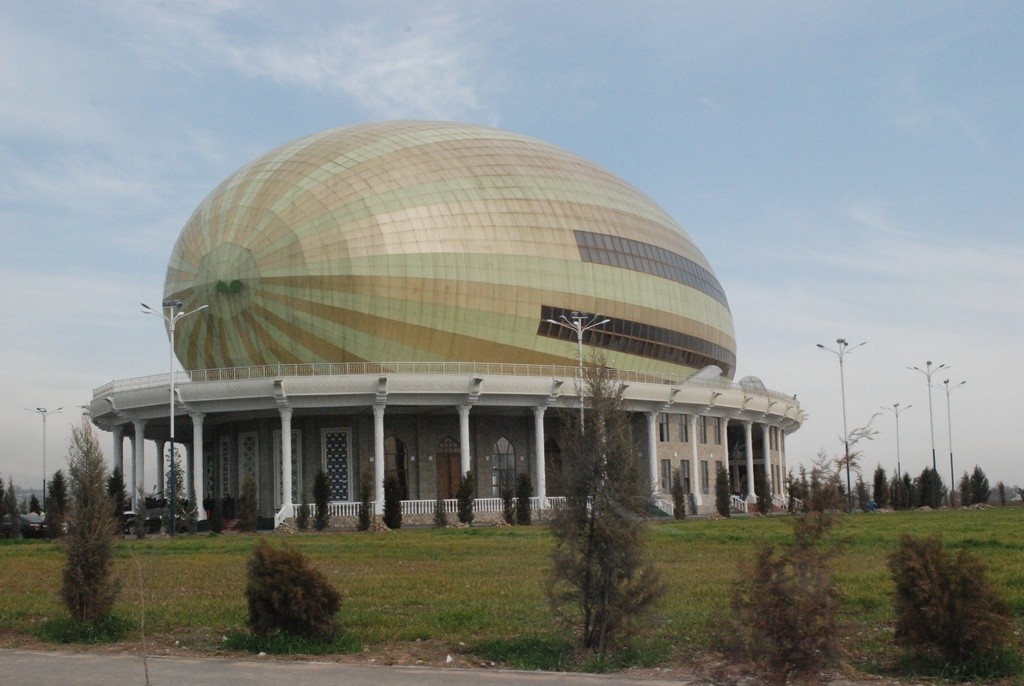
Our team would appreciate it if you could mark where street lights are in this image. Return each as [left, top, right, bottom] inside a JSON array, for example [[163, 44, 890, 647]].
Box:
[[906, 361, 953, 471], [817, 338, 868, 511], [929, 379, 967, 490], [879, 402, 913, 481], [141, 301, 209, 538], [25, 407, 63, 513], [544, 310, 611, 438]]
